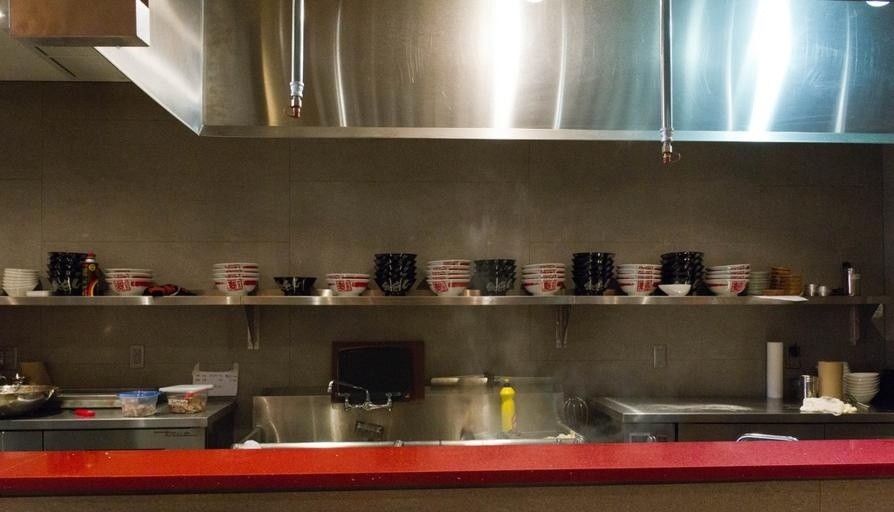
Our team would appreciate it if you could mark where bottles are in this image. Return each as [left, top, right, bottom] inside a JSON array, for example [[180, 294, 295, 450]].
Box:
[[498, 378, 519, 435]]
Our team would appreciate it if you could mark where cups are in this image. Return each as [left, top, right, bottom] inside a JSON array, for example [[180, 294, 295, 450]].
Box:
[[801, 359, 843, 403], [842, 267, 861, 295]]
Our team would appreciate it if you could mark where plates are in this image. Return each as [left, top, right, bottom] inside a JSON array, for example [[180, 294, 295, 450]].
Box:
[[1, 265, 40, 296]]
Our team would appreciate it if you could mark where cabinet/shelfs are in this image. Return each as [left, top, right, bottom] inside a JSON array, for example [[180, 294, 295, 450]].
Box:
[[590, 409, 894, 442], [0, 411, 234, 450]]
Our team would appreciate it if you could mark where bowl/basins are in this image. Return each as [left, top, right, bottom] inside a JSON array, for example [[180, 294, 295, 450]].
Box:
[[104, 266, 151, 296], [211, 262, 260, 294], [705, 264, 750, 297], [618, 262, 662, 296], [475, 258, 517, 297], [425, 259, 473, 297], [657, 253, 705, 296], [569, 253, 617, 295], [46, 250, 88, 295], [843, 361, 880, 403], [519, 263, 568, 296], [1, 384, 59, 418], [272, 276, 318, 295], [324, 272, 371, 296], [369, 253, 419, 297], [748, 266, 803, 297]]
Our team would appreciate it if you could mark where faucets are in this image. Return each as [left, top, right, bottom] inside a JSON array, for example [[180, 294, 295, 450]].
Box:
[[326, 380, 375, 414]]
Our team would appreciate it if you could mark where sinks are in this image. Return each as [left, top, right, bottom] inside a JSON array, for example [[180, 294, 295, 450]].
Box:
[[233, 374, 585, 451]]
